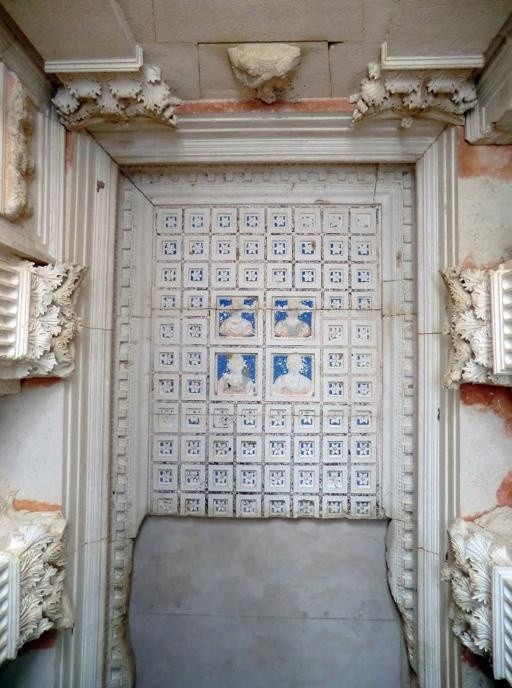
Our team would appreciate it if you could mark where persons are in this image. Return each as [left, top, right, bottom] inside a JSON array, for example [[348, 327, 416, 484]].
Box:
[[274, 355, 312, 396], [214, 352, 256, 397], [275, 300, 311, 339], [219, 298, 257, 337]]
